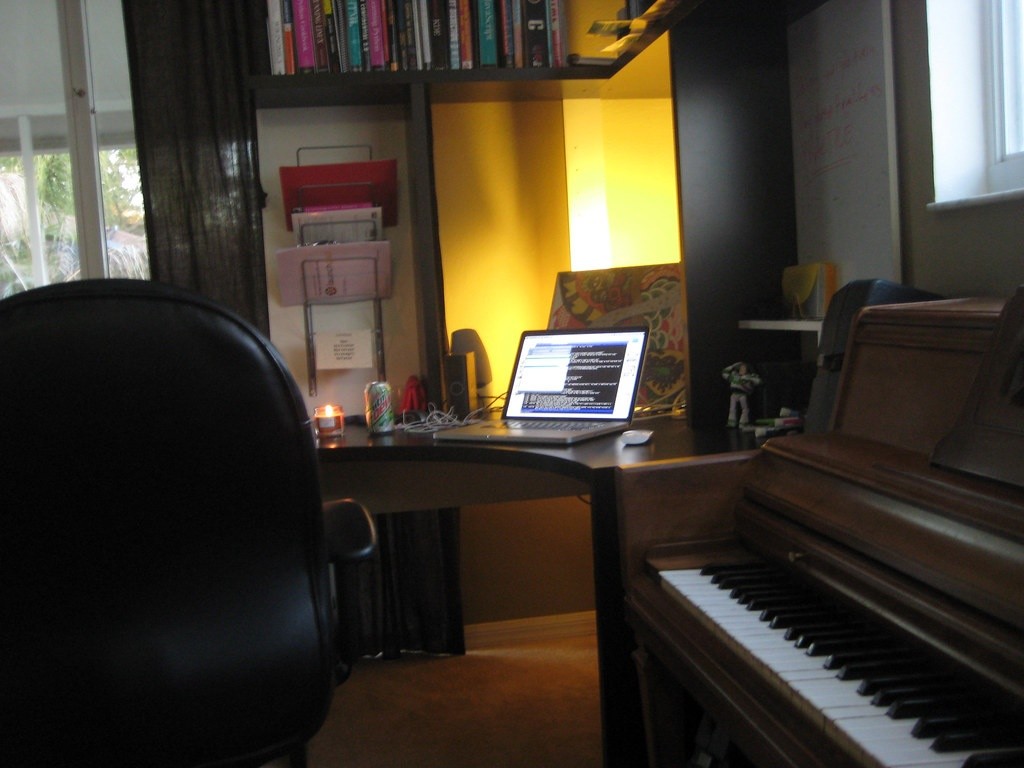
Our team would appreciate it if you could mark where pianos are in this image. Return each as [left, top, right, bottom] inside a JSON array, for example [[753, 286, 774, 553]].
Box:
[[591, 292, 1023, 768]]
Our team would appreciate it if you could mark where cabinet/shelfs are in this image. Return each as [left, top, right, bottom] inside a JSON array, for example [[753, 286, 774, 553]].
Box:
[[247, 0, 803, 428]]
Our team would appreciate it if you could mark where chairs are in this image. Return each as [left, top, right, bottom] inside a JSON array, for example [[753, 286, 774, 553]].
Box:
[[320, 500, 381, 687], [0, 280, 336, 768]]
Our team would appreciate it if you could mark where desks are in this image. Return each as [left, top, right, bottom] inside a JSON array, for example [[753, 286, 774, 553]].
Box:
[[309, 421, 800, 768]]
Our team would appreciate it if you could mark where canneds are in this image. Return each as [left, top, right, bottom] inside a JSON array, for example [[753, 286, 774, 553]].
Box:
[[364, 381, 394, 433]]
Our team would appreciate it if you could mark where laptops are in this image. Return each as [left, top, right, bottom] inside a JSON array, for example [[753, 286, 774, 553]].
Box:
[[433, 326, 651, 443]]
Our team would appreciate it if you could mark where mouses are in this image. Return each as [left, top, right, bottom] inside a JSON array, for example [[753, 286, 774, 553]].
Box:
[[621, 430, 654, 445]]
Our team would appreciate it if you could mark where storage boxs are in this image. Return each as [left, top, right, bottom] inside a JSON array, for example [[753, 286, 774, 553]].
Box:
[[782, 262, 836, 319]]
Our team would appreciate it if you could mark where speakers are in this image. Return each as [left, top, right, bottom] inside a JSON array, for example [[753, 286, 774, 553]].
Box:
[[449, 328, 492, 387], [443, 351, 478, 420]]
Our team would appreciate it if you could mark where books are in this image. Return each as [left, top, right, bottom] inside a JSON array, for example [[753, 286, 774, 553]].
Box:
[[264, 0, 569, 74]]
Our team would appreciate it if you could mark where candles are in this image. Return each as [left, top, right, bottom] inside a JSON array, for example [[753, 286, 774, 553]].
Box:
[[315, 405, 345, 437]]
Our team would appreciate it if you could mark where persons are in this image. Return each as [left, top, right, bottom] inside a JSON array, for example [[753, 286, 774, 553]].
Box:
[[722, 362, 763, 430]]
